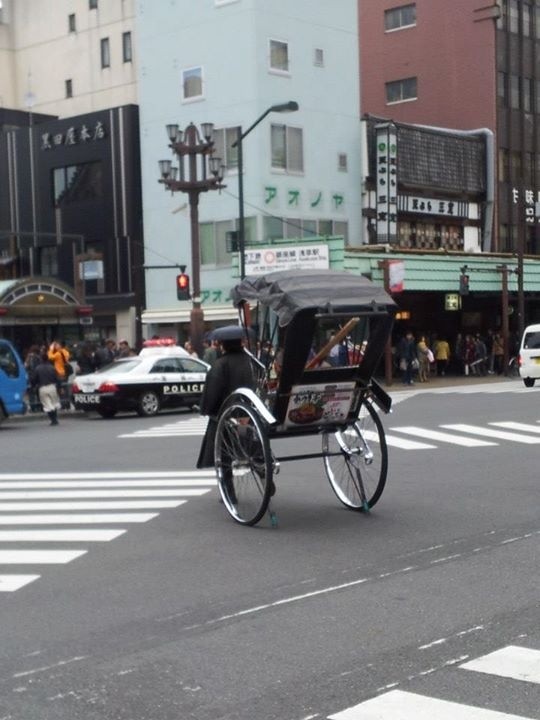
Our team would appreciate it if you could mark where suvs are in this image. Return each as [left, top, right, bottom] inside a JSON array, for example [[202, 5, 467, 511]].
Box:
[[0, 339, 29, 423], [518, 324, 540, 387]]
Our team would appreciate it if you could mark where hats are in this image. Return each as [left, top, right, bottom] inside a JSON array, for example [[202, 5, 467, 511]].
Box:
[[208, 325, 254, 338]]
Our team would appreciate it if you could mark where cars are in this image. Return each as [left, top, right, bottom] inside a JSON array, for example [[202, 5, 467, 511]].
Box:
[[69, 340, 212, 418]]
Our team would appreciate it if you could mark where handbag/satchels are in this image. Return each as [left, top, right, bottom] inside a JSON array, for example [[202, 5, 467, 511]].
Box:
[[63, 362, 73, 376], [427, 349, 434, 362]]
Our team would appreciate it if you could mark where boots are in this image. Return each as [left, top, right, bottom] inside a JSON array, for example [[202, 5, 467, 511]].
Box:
[[48, 409, 59, 425]]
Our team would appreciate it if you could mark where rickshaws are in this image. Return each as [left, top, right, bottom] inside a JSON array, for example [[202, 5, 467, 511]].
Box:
[[192, 268, 398, 529]]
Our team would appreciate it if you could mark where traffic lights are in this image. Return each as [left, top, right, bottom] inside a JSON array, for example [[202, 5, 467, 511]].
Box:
[[176, 274, 191, 301], [459, 275, 469, 295]]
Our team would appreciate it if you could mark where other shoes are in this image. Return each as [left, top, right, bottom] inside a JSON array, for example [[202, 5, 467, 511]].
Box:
[[270, 480, 276, 496], [218, 498, 237, 504]]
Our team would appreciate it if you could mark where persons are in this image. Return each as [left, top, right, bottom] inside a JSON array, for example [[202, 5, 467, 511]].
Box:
[[243, 340, 274, 379], [202, 339, 223, 365], [197, 339, 276, 503], [401, 332, 450, 384], [185, 341, 197, 357], [25, 340, 144, 425], [456, 334, 487, 376], [488, 329, 519, 375], [330, 335, 368, 367]]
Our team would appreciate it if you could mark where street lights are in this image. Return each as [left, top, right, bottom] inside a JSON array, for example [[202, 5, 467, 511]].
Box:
[[158, 121, 228, 361], [232, 101, 299, 283]]
